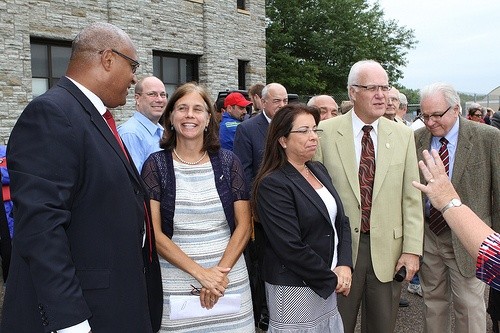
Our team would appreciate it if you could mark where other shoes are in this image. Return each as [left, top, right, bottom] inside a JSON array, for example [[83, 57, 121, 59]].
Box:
[[255, 313, 269, 331], [398, 299, 409, 306]]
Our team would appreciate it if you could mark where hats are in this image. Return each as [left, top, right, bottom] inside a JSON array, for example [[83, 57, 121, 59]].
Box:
[[224, 93, 253, 107]]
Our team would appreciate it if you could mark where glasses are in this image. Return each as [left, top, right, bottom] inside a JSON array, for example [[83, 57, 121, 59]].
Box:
[[190, 284, 202, 296], [352, 84, 392, 92], [137, 91, 166, 98], [99, 48, 140, 74], [388, 98, 399, 103], [473, 114, 483, 118], [289, 127, 323, 137], [419, 106, 451, 122]]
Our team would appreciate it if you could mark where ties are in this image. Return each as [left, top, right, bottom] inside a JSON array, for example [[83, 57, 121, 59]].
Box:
[[358, 125, 376, 234], [429, 137, 451, 236], [103, 109, 153, 264]]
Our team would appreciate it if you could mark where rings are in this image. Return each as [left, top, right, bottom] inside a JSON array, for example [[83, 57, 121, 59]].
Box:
[[428, 178, 433, 184], [344, 282, 348, 286], [210, 298, 216, 302]]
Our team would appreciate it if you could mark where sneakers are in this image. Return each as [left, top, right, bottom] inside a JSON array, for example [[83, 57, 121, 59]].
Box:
[[407, 283, 423, 296]]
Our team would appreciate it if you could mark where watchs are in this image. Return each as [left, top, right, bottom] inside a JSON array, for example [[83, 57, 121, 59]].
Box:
[[442, 198, 464, 214]]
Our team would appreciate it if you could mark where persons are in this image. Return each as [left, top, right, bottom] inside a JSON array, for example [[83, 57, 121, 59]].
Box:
[[413, 147, 500, 290], [1, 22, 163, 333], [464, 101, 500, 128], [143, 83, 259, 332], [252, 101, 354, 332], [214, 77, 345, 155], [120, 74, 172, 174], [382, 84, 431, 307], [411, 84, 500, 333], [309, 59, 425, 332], [235, 82, 290, 331]]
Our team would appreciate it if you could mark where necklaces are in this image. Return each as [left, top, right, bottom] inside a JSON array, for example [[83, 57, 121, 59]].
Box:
[[173, 146, 207, 165], [305, 165, 309, 179]]
